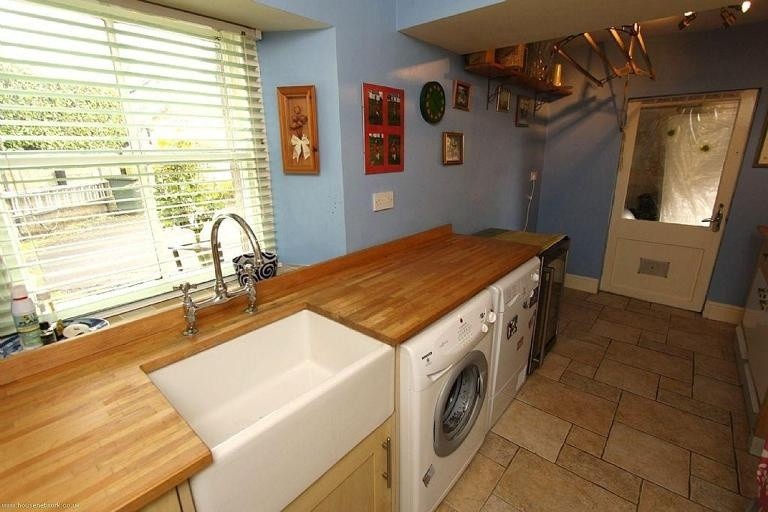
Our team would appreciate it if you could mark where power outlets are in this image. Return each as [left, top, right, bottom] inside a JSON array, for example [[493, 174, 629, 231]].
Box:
[[373, 190, 394, 211], [530, 171, 537, 181]]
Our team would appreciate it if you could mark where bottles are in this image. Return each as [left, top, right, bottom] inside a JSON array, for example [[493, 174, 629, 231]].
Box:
[[10, 285, 44, 352]]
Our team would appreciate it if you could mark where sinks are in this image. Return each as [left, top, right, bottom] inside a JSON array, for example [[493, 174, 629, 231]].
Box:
[[145, 308, 396, 512]]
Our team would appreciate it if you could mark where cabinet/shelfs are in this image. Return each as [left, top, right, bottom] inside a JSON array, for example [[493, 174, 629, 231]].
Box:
[[282, 415, 406, 512], [529, 236, 571, 375], [736, 225, 768, 428], [134, 482, 214, 512]]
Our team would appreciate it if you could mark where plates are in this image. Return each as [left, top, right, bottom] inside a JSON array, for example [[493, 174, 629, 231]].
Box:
[[0, 317, 109, 360]]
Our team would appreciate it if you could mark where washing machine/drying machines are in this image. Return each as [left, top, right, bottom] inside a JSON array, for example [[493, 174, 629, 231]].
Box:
[[399, 287, 495, 511]]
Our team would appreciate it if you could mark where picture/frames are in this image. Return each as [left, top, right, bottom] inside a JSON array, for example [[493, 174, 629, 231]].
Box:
[[453, 79, 473, 112], [752, 108, 768, 168], [441, 131, 464, 165], [362, 81, 405, 174], [497, 86, 511, 112], [515, 94, 532, 128], [276, 85, 321, 175]]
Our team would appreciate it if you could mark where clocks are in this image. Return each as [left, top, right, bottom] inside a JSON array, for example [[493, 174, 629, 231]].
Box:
[[419, 81, 445, 124]]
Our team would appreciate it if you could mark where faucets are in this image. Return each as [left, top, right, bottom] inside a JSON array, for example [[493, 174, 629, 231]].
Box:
[[209, 210, 265, 294]]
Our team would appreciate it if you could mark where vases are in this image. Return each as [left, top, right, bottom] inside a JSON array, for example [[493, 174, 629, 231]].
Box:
[[232, 251, 279, 287]]
